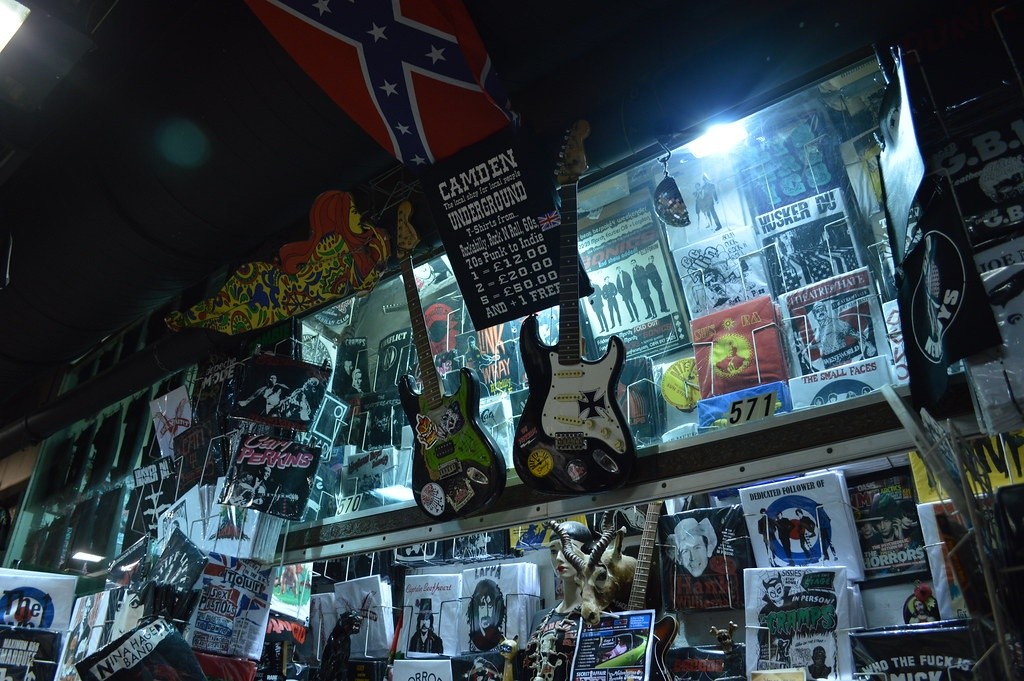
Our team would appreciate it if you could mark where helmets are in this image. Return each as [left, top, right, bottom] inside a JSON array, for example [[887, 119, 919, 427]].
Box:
[[871, 494, 902, 519]]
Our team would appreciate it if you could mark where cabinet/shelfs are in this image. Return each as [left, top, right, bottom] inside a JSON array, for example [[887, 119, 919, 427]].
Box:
[[1, 35, 1013, 681]]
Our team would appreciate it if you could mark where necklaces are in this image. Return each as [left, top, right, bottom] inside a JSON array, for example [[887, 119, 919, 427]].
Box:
[[529, 604, 582, 681]]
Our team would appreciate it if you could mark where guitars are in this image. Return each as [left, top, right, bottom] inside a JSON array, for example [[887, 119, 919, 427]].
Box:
[[391, 209, 508, 521], [510, 112, 640, 500], [589, 501, 679, 681]]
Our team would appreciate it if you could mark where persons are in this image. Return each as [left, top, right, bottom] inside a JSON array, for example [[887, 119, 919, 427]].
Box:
[[409, 598, 443, 655], [852, 493, 927, 575], [664, 517, 736, 610], [465, 579, 508, 652], [759, 505, 839, 567], [909, 599, 935, 624], [291, 335, 487, 453], [57, 596, 96, 681], [520, 521, 612, 681], [693, 172, 722, 232], [807, 646, 832, 678], [587, 256, 671, 333]]
